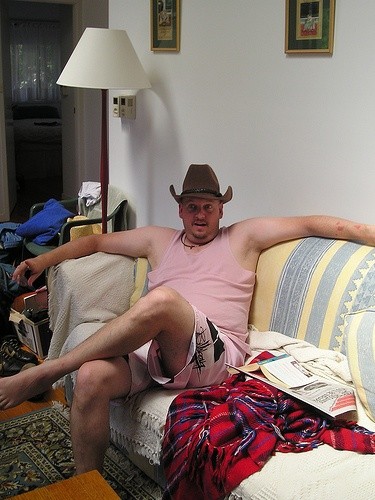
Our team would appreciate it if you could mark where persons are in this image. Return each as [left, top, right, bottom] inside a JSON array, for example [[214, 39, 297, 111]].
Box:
[[0, 164, 375, 476]]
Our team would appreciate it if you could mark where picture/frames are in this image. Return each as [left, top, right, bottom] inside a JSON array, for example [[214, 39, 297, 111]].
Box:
[[151, 0, 181, 51], [285, 0, 336, 53]]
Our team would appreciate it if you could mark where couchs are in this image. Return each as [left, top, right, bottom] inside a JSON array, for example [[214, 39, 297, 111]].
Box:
[[44, 237, 375, 500]]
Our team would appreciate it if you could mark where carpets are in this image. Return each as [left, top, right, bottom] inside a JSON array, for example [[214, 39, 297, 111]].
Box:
[[0, 404, 164, 500]]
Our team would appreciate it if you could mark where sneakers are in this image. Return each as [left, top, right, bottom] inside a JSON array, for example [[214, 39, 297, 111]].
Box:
[[0, 335, 39, 364], [0, 350, 25, 372]]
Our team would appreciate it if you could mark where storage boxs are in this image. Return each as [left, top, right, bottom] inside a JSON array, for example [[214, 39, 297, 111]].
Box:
[[9, 291, 53, 360]]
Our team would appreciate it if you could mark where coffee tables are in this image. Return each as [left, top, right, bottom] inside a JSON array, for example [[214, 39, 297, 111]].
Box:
[[6, 469, 122, 500]]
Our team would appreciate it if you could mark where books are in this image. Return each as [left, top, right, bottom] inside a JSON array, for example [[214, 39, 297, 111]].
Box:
[[226, 355, 317, 389]]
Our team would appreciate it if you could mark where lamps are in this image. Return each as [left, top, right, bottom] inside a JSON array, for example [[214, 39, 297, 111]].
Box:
[[56, 27, 152, 231]]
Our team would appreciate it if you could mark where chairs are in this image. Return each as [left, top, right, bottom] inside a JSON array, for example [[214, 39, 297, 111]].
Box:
[[20, 185, 128, 300]]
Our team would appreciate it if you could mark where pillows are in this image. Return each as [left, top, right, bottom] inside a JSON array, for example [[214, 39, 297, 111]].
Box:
[[344, 306, 375, 423]]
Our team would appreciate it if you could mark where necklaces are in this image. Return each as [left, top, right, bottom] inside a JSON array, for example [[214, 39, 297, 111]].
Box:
[[181, 229, 219, 250]]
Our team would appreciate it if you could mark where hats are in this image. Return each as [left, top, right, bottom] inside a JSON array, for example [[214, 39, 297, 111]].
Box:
[[170, 163, 233, 205]]
